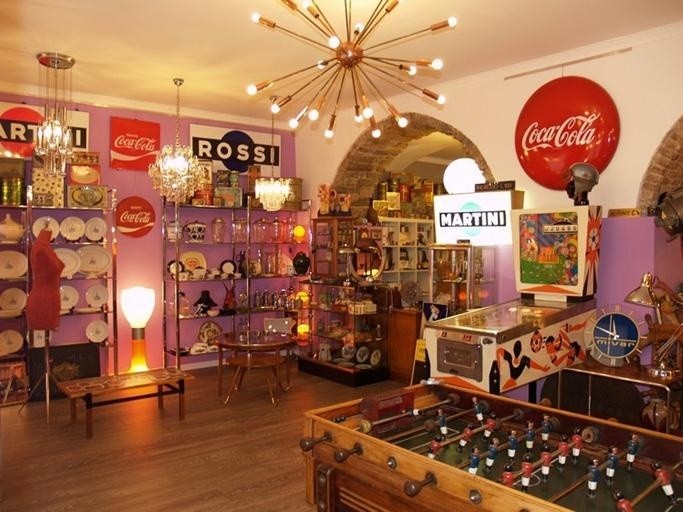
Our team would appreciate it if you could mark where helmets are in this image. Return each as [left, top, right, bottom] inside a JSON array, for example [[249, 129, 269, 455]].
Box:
[[567, 162, 600, 200]]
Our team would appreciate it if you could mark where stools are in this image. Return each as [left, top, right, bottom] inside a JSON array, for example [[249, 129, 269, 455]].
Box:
[[222, 353, 286, 409]]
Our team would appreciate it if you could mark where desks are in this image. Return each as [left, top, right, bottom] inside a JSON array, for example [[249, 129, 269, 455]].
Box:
[[303, 377, 683, 512], [423, 297, 596, 395], [556, 360, 680, 436], [57, 367, 195, 441], [212, 330, 298, 401]]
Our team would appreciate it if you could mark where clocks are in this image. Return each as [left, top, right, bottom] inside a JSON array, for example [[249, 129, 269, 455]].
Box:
[[583, 303, 648, 367]]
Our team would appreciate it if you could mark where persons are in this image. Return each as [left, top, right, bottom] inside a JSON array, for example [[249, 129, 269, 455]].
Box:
[[546, 322, 586, 368], [497, 340, 552, 390]]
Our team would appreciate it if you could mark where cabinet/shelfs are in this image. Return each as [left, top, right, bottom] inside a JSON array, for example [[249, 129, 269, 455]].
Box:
[[0, 186, 28, 409], [245, 196, 312, 359], [430, 244, 484, 314], [27, 186, 118, 404], [160, 195, 250, 391], [296, 278, 389, 387], [311, 217, 355, 284], [356, 215, 433, 308]]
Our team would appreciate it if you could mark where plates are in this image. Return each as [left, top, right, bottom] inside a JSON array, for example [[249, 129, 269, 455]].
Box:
[[341, 344, 356, 358], [86, 320, 109, 343], [32, 216, 60, 241], [26, 330, 52, 348], [0, 329, 23, 353], [76, 246, 112, 275], [197, 321, 223, 342], [60, 310, 69, 315], [180, 251, 207, 280], [355, 365, 371, 369], [60, 216, 85, 241], [85, 217, 106, 241], [0, 310, 22, 318], [333, 358, 348, 363], [54, 248, 80, 278], [60, 286, 78, 309], [338, 362, 354, 368], [220, 260, 237, 273], [86, 285, 108, 307], [356, 346, 370, 364], [0, 288, 27, 310], [371, 349, 382, 365], [74, 308, 101, 314], [167, 260, 184, 274], [0, 250, 28, 279]]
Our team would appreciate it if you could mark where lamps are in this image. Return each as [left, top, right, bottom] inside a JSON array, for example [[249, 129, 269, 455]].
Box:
[[147, 79, 207, 206], [243, 0, 457, 140], [254, 95, 293, 212], [35, 52, 75, 179], [623, 272, 680, 377], [120, 286, 156, 373], [655, 192, 682, 237]]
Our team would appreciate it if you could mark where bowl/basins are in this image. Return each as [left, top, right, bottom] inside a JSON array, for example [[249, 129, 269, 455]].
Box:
[[170, 347, 189, 357], [207, 310, 219, 317]]
